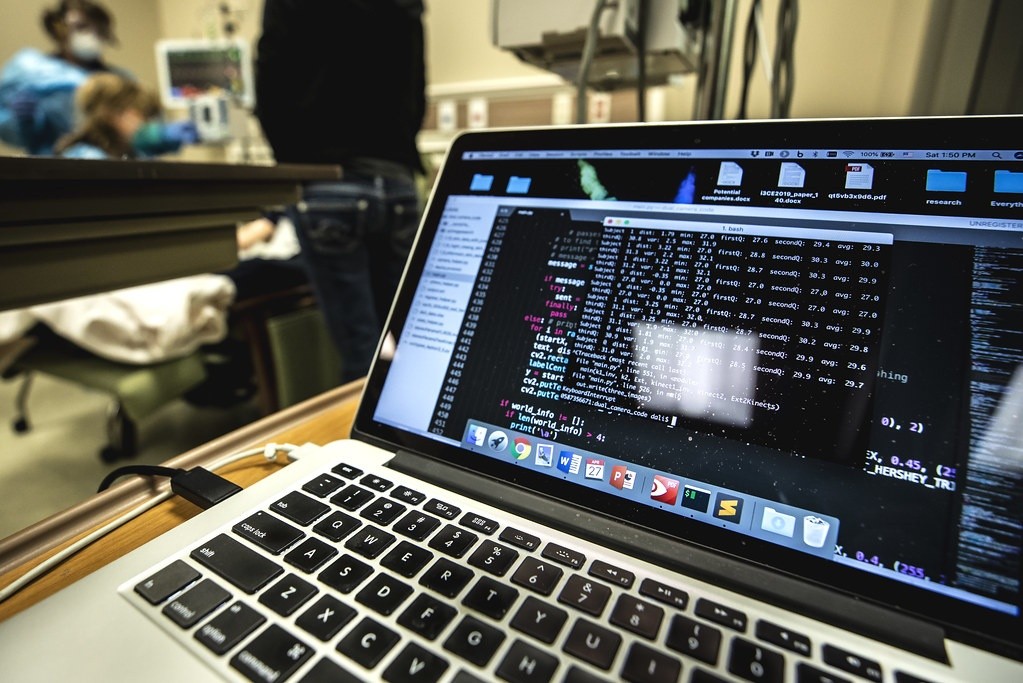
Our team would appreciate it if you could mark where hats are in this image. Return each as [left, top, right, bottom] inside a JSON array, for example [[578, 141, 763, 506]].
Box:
[[44, 0, 116, 44]]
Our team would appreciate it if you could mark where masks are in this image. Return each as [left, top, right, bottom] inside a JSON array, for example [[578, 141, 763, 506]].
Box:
[[67, 30, 101, 61]]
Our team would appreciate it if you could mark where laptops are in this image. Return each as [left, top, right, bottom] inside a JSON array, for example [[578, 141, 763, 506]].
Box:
[[0, 113, 1023, 683]]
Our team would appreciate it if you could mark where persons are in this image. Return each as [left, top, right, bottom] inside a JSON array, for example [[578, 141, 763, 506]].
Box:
[[185, 207, 308, 408], [0, 0, 198, 158], [253, 0, 428, 385], [52, 71, 160, 160]]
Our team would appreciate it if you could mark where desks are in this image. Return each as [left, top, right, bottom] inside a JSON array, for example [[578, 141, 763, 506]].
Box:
[[0, 156, 346, 419]]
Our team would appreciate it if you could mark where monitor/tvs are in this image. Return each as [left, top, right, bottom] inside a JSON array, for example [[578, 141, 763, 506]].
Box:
[[156, 37, 258, 114]]
